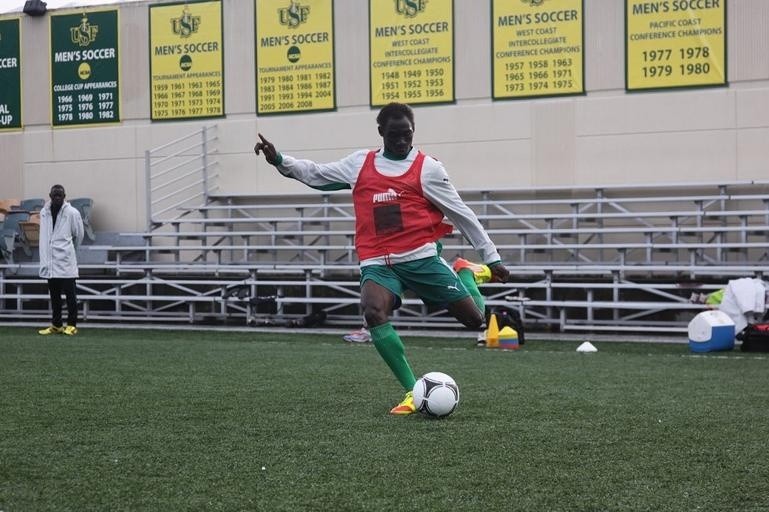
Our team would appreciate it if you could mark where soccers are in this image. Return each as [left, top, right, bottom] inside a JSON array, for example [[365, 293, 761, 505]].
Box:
[[413, 372, 459, 418]]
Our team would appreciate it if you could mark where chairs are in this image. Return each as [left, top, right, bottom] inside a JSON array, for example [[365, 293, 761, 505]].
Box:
[[0, 197, 95, 262]]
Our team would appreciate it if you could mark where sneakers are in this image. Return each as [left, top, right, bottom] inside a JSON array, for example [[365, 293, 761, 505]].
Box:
[[389, 390, 416, 415], [342, 327, 372, 342], [452, 258, 492, 285], [63, 326, 77, 336], [37, 326, 64, 335]]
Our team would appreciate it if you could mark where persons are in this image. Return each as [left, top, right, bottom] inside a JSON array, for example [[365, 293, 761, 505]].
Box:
[[36, 184, 85, 339], [251, 101, 513, 417]]
